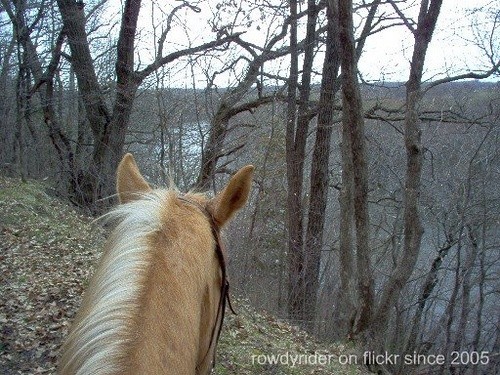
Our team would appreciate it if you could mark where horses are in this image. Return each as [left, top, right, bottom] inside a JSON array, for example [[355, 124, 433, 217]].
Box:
[[53, 151, 256, 375]]
